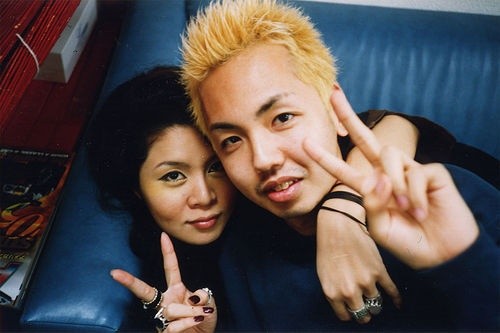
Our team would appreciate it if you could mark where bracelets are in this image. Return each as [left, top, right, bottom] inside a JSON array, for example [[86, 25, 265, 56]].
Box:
[[316, 205, 367, 229], [320, 191, 366, 211], [328, 182, 347, 193]]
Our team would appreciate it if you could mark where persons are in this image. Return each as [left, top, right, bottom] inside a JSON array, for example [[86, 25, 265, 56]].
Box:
[[80, 55, 500, 333], [177, 1, 500, 331]]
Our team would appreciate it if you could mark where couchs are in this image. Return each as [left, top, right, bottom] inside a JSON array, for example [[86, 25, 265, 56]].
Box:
[[22, 0, 500, 333]]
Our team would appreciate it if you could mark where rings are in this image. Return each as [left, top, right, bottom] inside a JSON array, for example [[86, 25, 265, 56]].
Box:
[[141, 287, 163, 309], [346, 303, 372, 322], [200, 287, 213, 306], [152, 307, 171, 330], [362, 292, 383, 310]]
